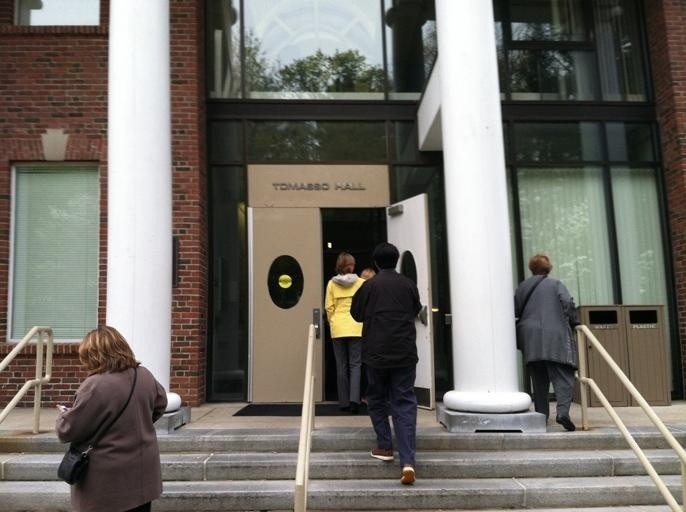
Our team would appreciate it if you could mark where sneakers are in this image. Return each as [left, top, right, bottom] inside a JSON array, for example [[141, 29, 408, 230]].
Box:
[[556, 414, 576, 431], [370, 447, 395, 461], [400, 463, 417, 485]]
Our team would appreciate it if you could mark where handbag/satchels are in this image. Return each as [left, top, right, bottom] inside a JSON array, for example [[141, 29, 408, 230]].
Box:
[[57, 445, 89, 485]]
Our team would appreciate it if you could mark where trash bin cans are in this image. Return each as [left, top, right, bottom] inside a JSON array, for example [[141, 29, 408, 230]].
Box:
[[574, 305, 671, 407]]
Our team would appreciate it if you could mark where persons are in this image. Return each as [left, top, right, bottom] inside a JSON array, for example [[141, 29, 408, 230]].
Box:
[[324, 251, 368, 415], [361, 267, 377, 411], [55, 326, 167, 512], [350, 242, 422, 485], [515, 254, 581, 431]]
